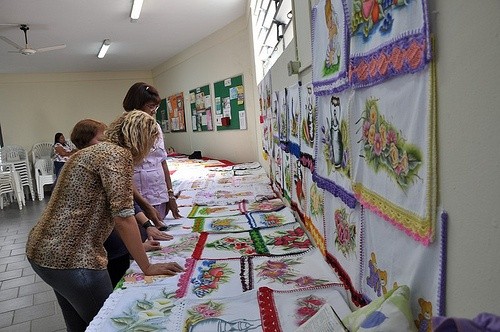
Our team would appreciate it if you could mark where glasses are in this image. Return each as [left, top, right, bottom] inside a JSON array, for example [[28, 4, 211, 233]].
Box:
[[150, 145, 156, 152], [146, 104, 160, 112]]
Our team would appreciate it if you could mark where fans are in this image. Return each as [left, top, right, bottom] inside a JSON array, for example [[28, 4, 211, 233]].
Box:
[[0, 24, 67, 56]]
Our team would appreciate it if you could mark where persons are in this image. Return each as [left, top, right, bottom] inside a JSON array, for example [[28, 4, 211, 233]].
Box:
[[123, 82, 183, 228], [25, 109, 184, 332], [71, 119, 173, 289], [49, 133, 77, 196]]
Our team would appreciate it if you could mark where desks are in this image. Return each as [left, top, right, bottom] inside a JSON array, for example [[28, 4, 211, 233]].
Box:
[[82, 153, 354, 332]]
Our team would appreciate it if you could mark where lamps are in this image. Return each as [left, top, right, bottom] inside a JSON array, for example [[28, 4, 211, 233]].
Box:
[[97, 39, 111, 59], [129, 0, 144, 23]]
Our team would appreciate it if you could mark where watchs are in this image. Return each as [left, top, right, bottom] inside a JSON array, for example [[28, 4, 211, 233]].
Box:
[[143, 219, 155, 229]]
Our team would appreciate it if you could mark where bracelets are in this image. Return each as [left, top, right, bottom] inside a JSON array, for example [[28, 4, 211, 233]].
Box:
[[168, 189, 173, 193]]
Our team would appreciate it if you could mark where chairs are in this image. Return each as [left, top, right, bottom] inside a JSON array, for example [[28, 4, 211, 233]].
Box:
[[0, 142, 56, 211]]
